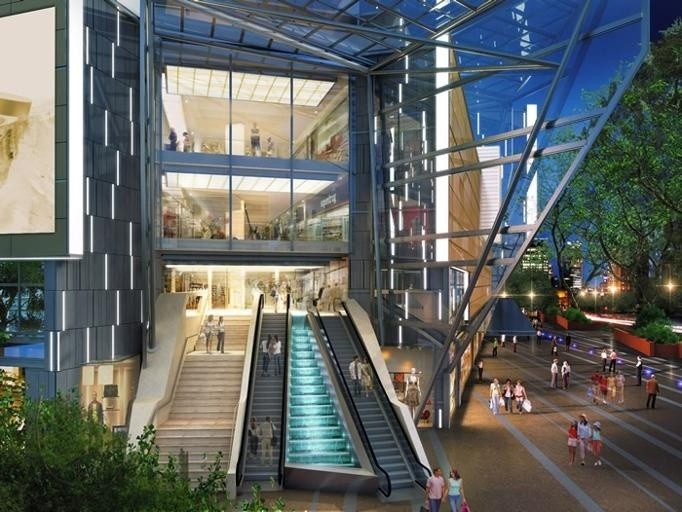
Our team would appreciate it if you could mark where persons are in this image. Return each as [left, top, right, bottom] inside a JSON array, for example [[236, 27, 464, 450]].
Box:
[[564, 332, 571, 352], [441, 469, 466, 511], [403, 368, 421, 421], [550, 336, 560, 355], [261, 333, 272, 377], [589, 370, 600, 406], [270, 282, 278, 313], [326, 284, 333, 311], [512, 336, 518, 353], [513, 379, 528, 414], [272, 333, 284, 377], [423, 467, 445, 512], [203, 313, 213, 354], [530, 317, 543, 331], [265, 135, 275, 158], [589, 421, 604, 466], [475, 357, 483, 384], [248, 414, 258, 458], [188, 129, 196, 153], [560, 360, 570, 391], [645, 373, 659, 409], [598, 372, 608, 405], [634, 354, 642, 386], [181, 131, 192, 153], [550, 358, 558, 388], [87, 390, 107, 454], [260, 415, 276, 466], [490, 376, 501, 415], [359, 356, 374, 397], [608, 349, 617, 373], [614, 370, 625, 405], [331, 283, 342, 311], [215, 315, 226, 353], [600, 348, 607, 372], [577, 413, 592, 465], [491, 337, 498, 358], [605, 371, 615, 406], [502, 378, 513, 414], [501, 334, 506, 347], [536, 329, 542, 345], [347, 355, 361, 397], [169, 127, 178, 151], [250, 121, 262, 157], [566, 419, 577, 464]]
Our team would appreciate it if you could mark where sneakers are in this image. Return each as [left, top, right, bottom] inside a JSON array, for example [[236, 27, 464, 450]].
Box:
[[581, 461, 601, 467]]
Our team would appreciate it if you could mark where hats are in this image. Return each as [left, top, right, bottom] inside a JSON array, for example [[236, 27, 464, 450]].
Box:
[[593, 421, 601, 429]]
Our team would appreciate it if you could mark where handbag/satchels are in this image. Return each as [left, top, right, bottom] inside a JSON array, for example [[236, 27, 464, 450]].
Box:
[[460, 504, 470, 512], [522, 401, 533, 413], [420, 505, 430, 512], [487, 398, 493, 409], [567, 438, 578, 447], [270, 437, 278, 447], [499, 397, 505, 408]]
